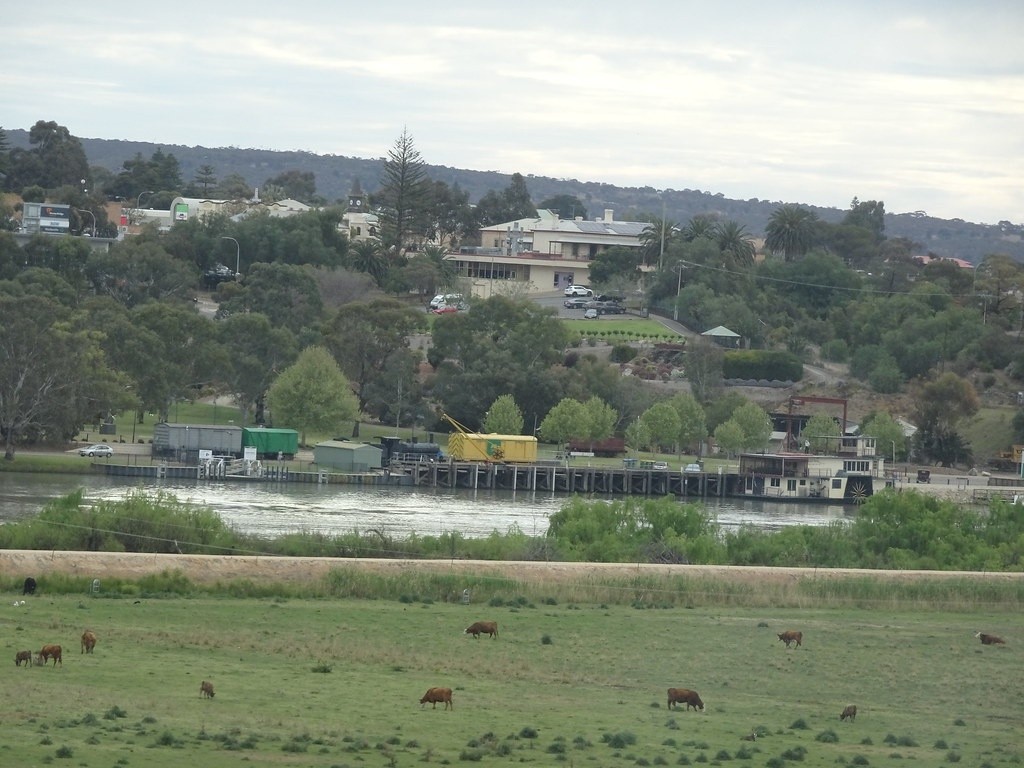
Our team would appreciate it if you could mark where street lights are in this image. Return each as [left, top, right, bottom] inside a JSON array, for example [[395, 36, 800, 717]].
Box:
[[136, 190, 155, 222], [222, 236, 240, 284], [80, 210, 96, 237]]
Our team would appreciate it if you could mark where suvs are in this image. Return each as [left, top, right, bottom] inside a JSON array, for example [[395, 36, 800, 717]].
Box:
[[590, 302, 626, 315], [565, 285, 594, 297]]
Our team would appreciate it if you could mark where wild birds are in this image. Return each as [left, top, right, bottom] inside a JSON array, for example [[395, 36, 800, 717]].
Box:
[[13, 600, 25, 607]]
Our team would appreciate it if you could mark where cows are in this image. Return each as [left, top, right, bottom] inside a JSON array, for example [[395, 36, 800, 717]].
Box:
[[35, 644, 62, 667], [840, 705, 856, 723], [777, 631, 802, 649], [419, 687, 453, 711], [975, 632, 1005, 645], [463, 622, 499, 639], [198, 681, 216, 699], [667, 688, 704, 712], [81, 631, 96, 654], [23, 577, 36, 595], [15, 650, 32, 667]]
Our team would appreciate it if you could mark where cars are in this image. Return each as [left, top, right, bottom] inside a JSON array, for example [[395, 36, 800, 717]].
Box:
[[432, 305, 457, 314], [652, 462, 668, 471], [684, 463, 700, 473], [430, 292, 466, 311], [583, 300, 596, 311], [79, 445, 114, 458], [584, 309, 597, 320], [564, 298, 589, 309], [593, 290, 625, 301]]
